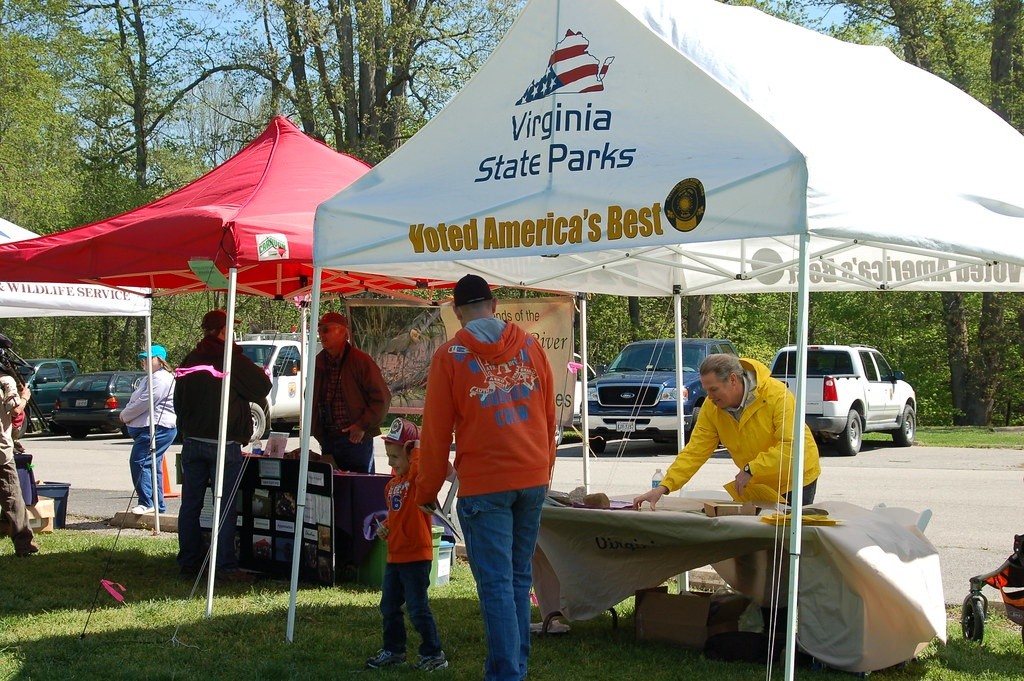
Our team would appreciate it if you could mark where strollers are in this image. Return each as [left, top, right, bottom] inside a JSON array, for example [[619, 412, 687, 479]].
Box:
[[959, 533, 1023, 643]]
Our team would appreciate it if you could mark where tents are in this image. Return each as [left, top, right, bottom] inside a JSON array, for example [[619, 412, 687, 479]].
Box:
[[0, 117, 574, 617], [0, 218, 159, 537], [286, 0, 1024, 681]]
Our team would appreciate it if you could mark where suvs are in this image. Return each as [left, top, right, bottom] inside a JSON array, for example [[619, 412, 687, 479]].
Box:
[[234, 339, 308, 443], [580, 338, 740, 453], [769, 343, 917, 456], [12, 358, 83, 417]]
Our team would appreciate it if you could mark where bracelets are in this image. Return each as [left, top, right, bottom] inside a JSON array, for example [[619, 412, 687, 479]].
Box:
[[21, 397, 28, 404]]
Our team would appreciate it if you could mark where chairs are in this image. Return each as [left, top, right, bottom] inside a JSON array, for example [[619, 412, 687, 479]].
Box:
[[872, 503, 932, 533], [439, 461, 459, 515]]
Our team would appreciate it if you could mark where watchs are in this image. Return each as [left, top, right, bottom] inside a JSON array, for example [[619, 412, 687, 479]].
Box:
[[744, 464, 753, 477]]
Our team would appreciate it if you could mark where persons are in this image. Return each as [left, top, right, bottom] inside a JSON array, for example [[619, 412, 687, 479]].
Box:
[[304, 313, 391, 474], [414, 274, 557, 681], [172, 311, 273, 586], [366, 418, 449, 673], [1, 370, 40, 556], [119, 344, 178, 515], [633, 354, 822, 647]]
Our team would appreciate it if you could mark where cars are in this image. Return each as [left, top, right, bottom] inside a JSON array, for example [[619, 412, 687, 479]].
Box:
[[554, 353, 597, 446], [50, 371, 147, 438]]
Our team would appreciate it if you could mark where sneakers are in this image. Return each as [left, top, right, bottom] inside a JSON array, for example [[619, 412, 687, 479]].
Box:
[[368, 648, 407, 669], [131, 505, 155, 515], [413, 650, 448, 672]]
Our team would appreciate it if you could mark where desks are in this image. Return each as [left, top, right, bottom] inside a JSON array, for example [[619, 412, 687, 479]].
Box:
[[333, 473, 393, 582], [533, 493, 946, 673]]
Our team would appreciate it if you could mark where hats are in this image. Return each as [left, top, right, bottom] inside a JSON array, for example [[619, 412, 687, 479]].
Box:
[[201, 309, 241, 332], [318, 312, 346, 326], [381, 418, 420, 447], [454, 274, 493, 306], [138, 345, 166, 361]]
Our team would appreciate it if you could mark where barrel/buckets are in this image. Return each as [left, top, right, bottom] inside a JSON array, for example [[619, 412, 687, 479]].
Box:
[[35, 482, 71, 529]]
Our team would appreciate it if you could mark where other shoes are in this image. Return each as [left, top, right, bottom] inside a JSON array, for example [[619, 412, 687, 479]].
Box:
[[16, 543, 39, 557]]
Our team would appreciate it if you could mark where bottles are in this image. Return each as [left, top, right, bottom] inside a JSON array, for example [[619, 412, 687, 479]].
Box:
[[652, 469, 665, 490], [252, 436, 261, 455]]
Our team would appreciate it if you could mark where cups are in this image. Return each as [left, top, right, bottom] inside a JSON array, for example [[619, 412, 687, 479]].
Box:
[[263, 432, 290, 458]]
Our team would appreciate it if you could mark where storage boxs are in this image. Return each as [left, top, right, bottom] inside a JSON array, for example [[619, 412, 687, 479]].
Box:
[[357, 525, 444, 590], [27, 495, 55, 533], [438, 540, 454, 587], [36, 482, 71, 529], [635, 586, 739, 650], [704, 500, 762, 518]]
